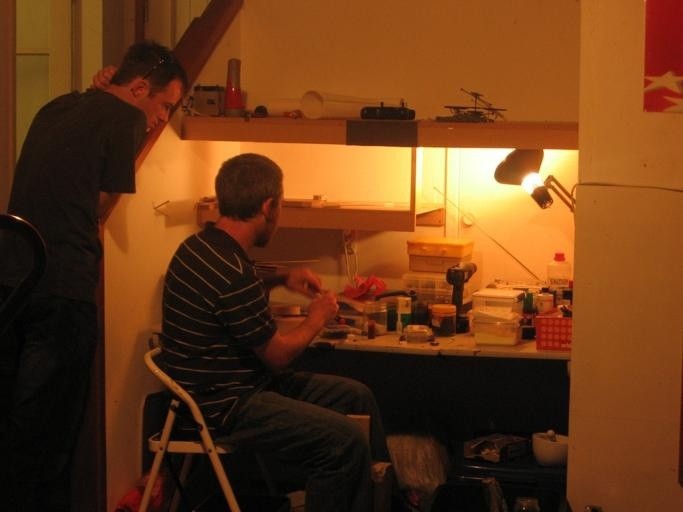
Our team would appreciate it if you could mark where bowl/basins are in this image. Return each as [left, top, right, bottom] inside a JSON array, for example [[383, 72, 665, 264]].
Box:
[[533, 431, 568, 469]]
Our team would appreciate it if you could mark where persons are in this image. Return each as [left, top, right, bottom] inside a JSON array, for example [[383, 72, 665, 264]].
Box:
[[158, 151, 402, 511], [0, 38, 190, 511]]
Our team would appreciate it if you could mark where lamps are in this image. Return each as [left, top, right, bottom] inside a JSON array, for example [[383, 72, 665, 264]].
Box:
[[491, 147, 576, 215]]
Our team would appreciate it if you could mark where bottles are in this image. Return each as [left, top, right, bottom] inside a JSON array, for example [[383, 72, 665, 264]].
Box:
[[547, 252, 572, 305], [363, 300, 388, 335], [430, 303, 458, 337], [534, 287, 554, 314]]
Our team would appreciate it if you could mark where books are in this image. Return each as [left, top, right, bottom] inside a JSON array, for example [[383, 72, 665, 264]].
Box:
[[281, 196, 330, 209]]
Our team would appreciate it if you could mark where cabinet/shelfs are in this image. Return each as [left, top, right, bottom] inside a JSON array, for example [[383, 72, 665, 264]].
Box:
[[182, 115, 579, 361]]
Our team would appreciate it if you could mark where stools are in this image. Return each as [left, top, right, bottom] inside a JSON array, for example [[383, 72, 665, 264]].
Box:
[[460, 438, 568, 512]]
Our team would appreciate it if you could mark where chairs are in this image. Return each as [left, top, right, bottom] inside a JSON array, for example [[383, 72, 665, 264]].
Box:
[[140, 348, 243, 512]]
